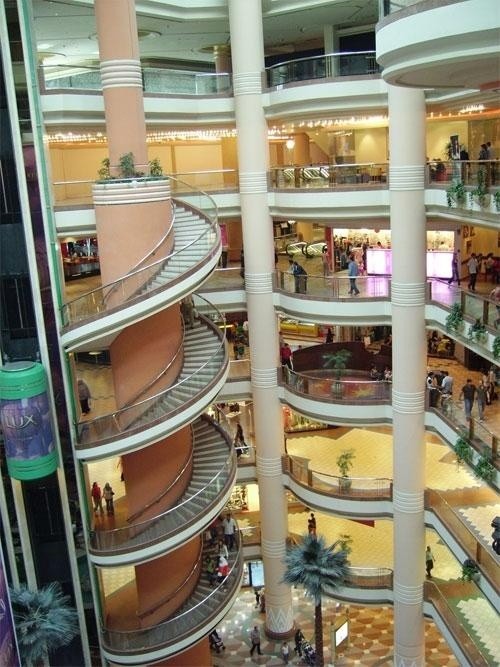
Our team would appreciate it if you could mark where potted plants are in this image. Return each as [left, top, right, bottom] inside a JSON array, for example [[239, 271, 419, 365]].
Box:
[[454, 438, 497, 482], [335, 448, 357, 491], [461, 565, 481, 587], [321, 349, 353, 399], [335, 534, 354, 566], [445, 184, 491, 218], [444, 302, 489, 347]]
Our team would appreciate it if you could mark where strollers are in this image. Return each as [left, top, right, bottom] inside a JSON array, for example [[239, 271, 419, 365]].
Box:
[[210, 629, 224, 652], [300, 638, 316, 665]]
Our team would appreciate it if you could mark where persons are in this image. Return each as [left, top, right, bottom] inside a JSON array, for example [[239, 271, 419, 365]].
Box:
[[464, 249, 496, 291], [293, 627, 305, 657], [292, 261, 308, 294], [232, 421, 247, 446], [248, 625, 264, 655], [425, 545, 437, 576], [180, 293, 197, 330], [280, 343, 295, 368], [259, 594, 266, 612], [77, 378, 93, 414], [201, 512, 237, 585], [427, 330, 499, 424], [425, 136, 498, 187], [346, 253, 360, 294], [487, 284, 500, 330], [447, 252, 461, 286], [377, 241, 385, 249], [101, 481, 115, 516], [321, 248, 331, 278], [280, 640, 290, 664], [208, 629, 219, 648], [253, 591, 260, 607], [370, 362, 392, 382], [307, 518, 313, 536], [90, 481, 104, 514], [310, 512, 316, 535], [278, 330, 286, 347], [287, 259, 300, 292], [324, 327, 335, 343], [238, 250, 246, 286], [232, 316, 249, 360]]
